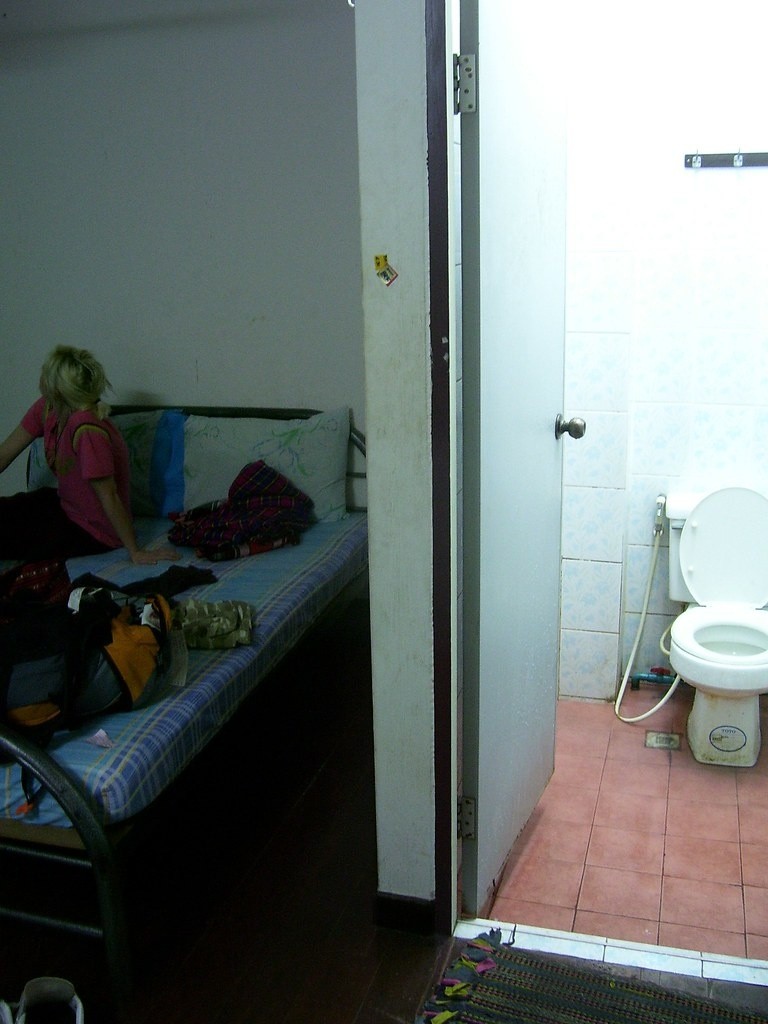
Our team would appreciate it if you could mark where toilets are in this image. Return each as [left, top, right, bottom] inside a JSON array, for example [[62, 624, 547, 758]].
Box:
[[666, 484, 767, 766]]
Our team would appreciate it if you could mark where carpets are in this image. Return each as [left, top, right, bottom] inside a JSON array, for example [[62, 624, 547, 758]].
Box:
[[407, 926, 764, 1024]]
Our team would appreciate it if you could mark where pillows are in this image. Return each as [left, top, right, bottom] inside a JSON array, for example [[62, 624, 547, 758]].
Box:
[[26, 409, 187, 522], [161, 405, 350, 525]]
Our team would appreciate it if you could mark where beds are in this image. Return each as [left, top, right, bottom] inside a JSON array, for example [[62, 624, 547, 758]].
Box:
[[1, 405, 369, 1024]]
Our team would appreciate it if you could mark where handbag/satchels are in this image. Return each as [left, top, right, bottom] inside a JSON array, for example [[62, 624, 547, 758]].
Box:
[[0, 590, 171, 764]]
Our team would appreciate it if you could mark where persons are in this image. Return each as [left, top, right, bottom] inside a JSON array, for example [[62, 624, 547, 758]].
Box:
[[0, 345, 183, 564]]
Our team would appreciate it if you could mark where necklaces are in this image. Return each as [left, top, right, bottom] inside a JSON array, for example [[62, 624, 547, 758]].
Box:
[[55, 416, 69, 457]]
